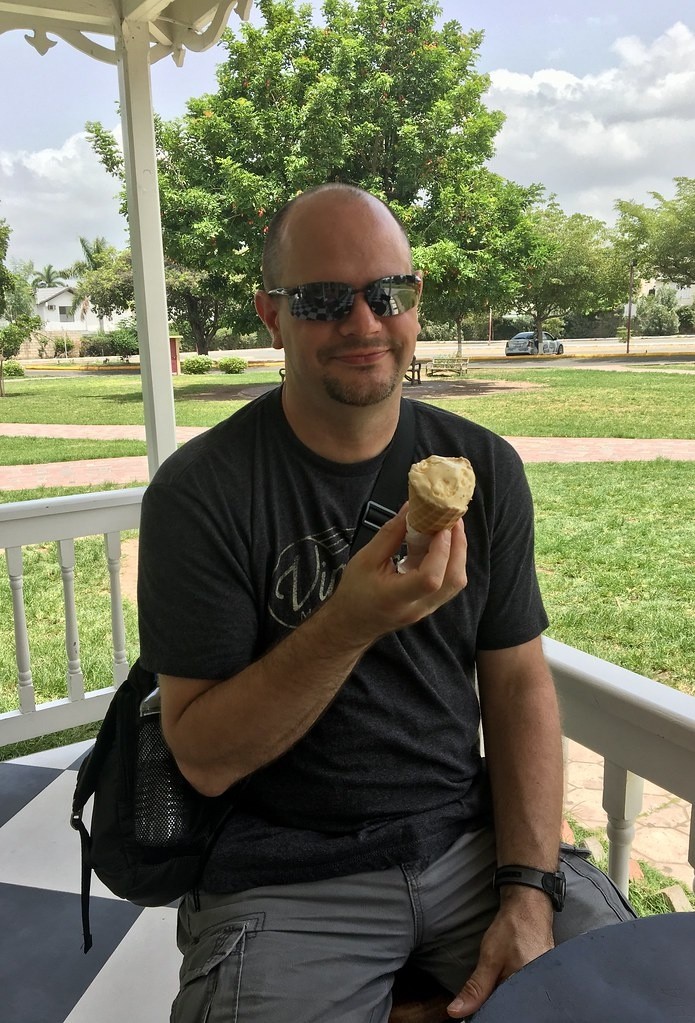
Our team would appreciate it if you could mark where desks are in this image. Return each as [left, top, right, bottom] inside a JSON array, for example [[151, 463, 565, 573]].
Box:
[[469, 912, 695, 1023]]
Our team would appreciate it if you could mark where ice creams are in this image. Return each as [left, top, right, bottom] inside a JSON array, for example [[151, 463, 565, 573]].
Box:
[[407, 454, 475, 536]]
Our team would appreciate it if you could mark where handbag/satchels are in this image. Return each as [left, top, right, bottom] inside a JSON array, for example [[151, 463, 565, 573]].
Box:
[[70, 655, 230, 954]]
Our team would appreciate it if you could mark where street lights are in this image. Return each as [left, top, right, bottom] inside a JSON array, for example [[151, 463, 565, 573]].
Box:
[[626, 258, 638, 354]]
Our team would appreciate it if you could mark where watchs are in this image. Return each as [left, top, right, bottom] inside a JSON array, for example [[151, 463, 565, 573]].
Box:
[[491, 863, 569, 913]]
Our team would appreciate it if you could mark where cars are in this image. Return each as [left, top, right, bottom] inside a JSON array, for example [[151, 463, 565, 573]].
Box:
[[505, 331, 564, 356]]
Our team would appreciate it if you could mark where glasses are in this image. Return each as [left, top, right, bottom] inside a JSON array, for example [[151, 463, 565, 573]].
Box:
[[267, 275, 422, 322]]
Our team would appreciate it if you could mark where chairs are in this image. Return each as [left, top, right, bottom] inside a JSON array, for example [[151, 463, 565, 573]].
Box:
[[405, 356, 421, 386]]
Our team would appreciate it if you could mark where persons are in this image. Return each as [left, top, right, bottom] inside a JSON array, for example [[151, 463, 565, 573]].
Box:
[[139, 183, 640, 1023]]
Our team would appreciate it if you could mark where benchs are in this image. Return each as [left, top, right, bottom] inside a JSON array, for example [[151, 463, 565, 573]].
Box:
[[426, 358, 469, 376]]
